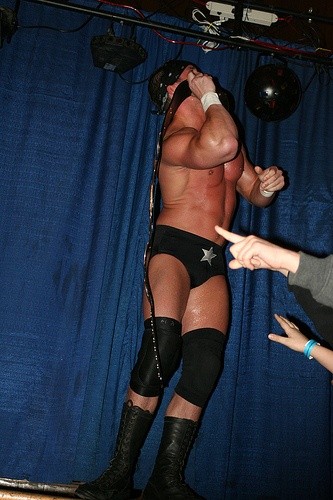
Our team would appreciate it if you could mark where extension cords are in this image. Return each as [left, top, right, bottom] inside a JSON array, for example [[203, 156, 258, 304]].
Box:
[[206, 1, 278, 27]]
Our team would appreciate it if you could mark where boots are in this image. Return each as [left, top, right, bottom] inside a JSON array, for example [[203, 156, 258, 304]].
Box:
[[141, 416, 205, 500], [76, 400, 155, 500]]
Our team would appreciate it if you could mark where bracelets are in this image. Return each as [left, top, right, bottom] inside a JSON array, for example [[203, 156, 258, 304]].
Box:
[[200, 92, 222, 113], [304, 339, 321, 359], [259, 182, 274, 198]]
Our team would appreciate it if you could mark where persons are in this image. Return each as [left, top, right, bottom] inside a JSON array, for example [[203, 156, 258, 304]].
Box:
[[73, 58, 286, 500], [268, 314, 333, 374], [214, 224, 333, 309]]
